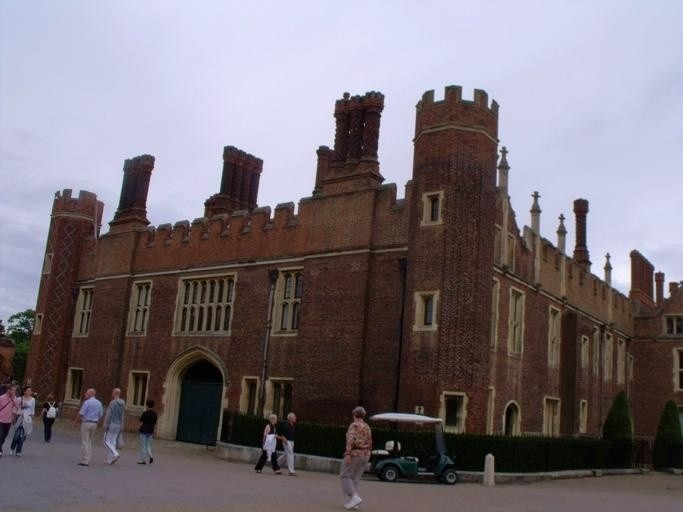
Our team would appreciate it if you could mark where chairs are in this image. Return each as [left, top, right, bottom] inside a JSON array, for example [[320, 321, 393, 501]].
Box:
[[371, 439, 394, 461], [396, 440, 419, 463]]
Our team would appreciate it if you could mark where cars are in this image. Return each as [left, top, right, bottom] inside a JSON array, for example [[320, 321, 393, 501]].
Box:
[[0, 383, 24, 397]]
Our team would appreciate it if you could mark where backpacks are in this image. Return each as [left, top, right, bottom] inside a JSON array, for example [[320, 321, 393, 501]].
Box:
[[45, 401, 56, 419]]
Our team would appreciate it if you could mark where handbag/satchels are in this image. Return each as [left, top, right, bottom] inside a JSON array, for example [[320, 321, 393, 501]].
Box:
[[116, 432, 124, 449]]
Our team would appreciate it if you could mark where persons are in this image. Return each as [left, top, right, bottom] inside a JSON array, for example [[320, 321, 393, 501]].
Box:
[[7, 386, 35, 457], [71, 389, 104, 468], [253, 413, 282, 475], [34, 392, 58, 442], [103, 387, 125, 465], [338, 407, 372, 510], [276, 412, 297, 476], [0, 385, 16, 457], [135, 400, 156, 465]]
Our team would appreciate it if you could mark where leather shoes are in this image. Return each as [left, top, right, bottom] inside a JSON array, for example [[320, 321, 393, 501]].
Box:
[[111, 454, 120, 464]]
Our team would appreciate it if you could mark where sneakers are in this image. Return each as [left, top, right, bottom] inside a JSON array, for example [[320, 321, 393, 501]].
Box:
[[344, 496, 362, 509], [137, 461, 145, 464]]
